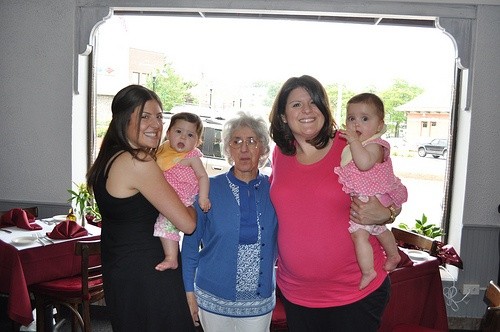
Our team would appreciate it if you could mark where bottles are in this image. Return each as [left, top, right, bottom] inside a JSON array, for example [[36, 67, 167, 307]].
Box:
[[67, 208, 77, 222]]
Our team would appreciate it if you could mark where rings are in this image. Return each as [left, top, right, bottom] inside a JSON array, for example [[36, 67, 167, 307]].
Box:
[[351, 210, 356, 217]]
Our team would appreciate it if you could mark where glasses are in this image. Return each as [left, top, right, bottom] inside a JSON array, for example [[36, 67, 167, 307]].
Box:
[[229, 138, 261, 149]]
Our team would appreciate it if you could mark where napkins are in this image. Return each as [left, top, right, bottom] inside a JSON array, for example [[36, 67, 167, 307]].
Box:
[[0, 208, 42, 231], [46, 220, 88, 240]]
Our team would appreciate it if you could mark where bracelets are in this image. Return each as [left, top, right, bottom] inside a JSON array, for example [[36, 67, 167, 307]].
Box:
[[386, 205, 397, 226]]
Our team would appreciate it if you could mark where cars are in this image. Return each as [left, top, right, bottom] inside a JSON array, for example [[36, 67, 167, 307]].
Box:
[[418, 139, 448, 160], [161, 111, 273, 179]]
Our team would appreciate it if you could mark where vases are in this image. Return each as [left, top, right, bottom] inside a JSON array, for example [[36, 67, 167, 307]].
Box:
[[80, 213, 88, 229]]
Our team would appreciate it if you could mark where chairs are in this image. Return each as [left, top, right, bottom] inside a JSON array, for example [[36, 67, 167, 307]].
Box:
[[31, 240, 103, 332], [391, 226, 436, 254], [483, 280, 500, 332]]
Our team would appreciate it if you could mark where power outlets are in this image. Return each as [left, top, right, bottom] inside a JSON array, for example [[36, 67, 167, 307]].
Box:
[[464, 283, 480, 295]]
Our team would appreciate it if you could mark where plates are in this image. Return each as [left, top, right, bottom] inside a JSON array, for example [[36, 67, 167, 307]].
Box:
[[53, 215, 68, 221], [404, 249, 429, 260], [11, 236, 37, 244]]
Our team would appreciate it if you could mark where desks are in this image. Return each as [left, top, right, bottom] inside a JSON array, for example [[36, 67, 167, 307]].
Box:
[[0, 214, 104, 332], [378, 246, 449, 332]]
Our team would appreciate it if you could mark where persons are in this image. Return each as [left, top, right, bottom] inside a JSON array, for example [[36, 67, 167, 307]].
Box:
[[153, 113, 211, 271], [266, 75, 402, 332], [182, 112, 280, 332], [334, 93, 408, 290], [87, 85, 204, 332]]
